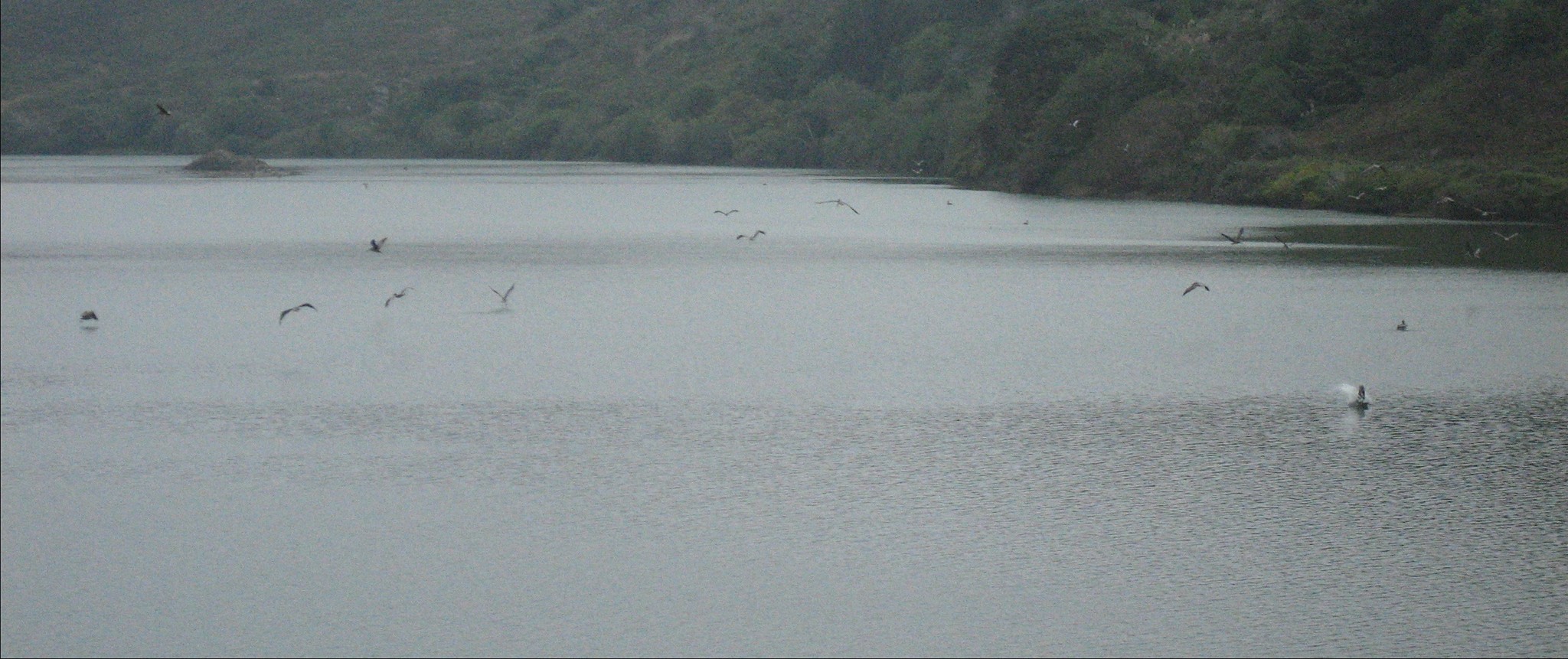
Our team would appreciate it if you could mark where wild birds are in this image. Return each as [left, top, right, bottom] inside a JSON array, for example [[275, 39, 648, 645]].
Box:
[[280, 303, 317, 321], [386, 287, 414, 305], [1397, 319, 1411, 331], [1356, 384, 1372, 411], [1276, 236, 1298, 253], [81, 310, 100, 321], [1220, 228, 1247, 246], [817, 196, 860, 219], [1345, 160, 1499, 220], [364, 237, 390, 253], [1465, 248, 1482, 259], [1182, 280, 1210, 295], [154, 103, 171, 117], [490, 284, 515, 304], [715, 209, 769, 243], [1495, 231, 1520, 242]]
